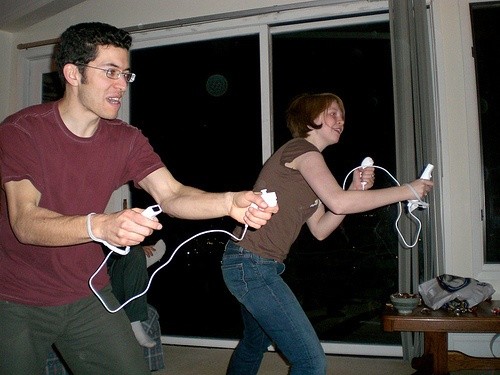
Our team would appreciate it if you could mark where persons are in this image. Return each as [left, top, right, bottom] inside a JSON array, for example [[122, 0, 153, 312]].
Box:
[[1, 22, 280, 375], [101, 238, 167, 348], [220, 93, 435, 375]]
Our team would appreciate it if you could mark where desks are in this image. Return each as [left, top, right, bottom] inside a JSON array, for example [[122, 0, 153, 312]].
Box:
[[383, 300, 500, 375]]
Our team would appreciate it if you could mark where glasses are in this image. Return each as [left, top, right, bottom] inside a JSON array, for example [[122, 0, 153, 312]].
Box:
[[77, 64, 136, 83]]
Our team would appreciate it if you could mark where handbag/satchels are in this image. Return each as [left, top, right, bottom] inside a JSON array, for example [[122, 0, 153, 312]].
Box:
[[417, 274, 496, 311]]
